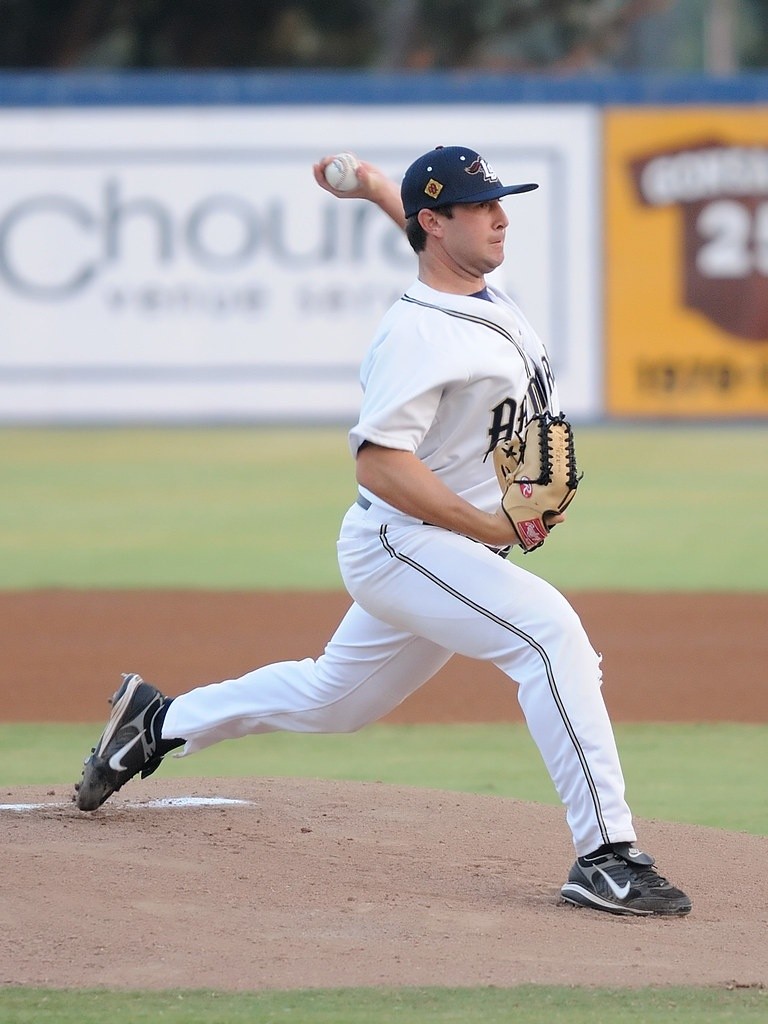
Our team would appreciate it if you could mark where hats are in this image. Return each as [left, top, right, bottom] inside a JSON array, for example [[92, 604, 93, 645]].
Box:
[[401, 146, 539, 219]]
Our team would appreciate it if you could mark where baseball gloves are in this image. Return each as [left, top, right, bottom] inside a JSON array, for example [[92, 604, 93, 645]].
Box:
[[491, 410, 580, 550]]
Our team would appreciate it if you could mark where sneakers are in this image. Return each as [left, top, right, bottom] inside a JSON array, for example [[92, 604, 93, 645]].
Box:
[[560, 845, 692, 917], [72, 672, 166, 811]]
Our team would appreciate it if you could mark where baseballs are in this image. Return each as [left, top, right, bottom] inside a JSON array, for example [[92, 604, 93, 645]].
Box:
[[325, 152, 362, 192]]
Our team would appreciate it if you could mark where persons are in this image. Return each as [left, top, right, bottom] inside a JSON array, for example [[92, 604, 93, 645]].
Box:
[[72, 148, 693, 917]]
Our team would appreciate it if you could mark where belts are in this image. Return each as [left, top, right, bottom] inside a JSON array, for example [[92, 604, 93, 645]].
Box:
[[356, 492, 514, 560]]
